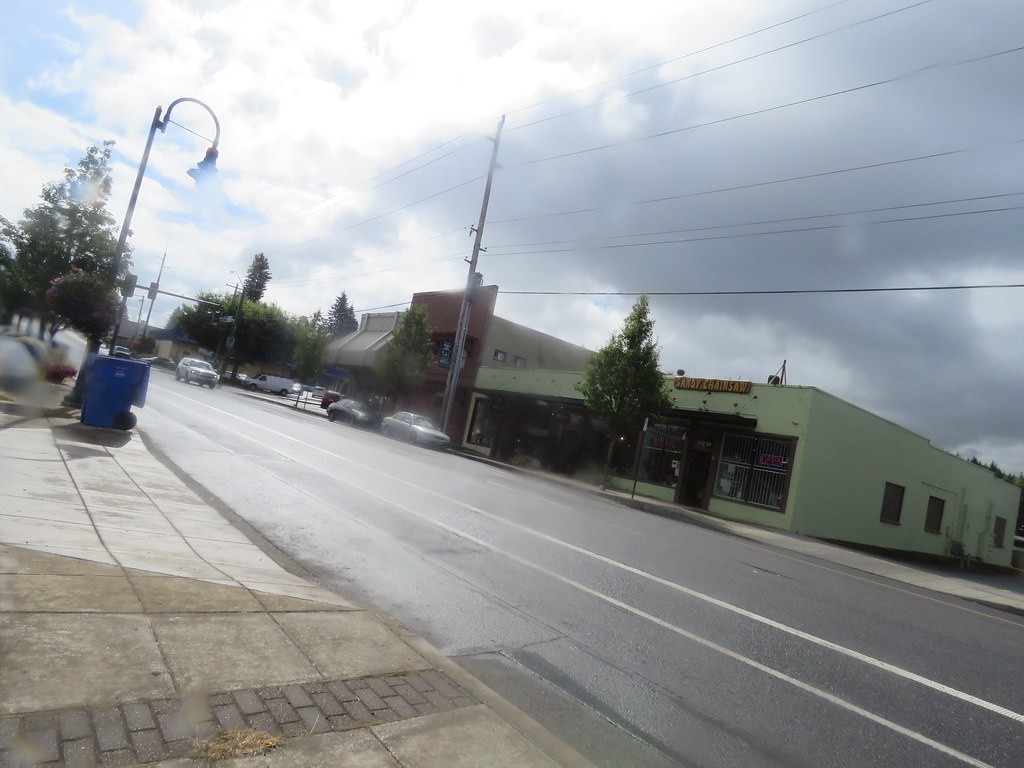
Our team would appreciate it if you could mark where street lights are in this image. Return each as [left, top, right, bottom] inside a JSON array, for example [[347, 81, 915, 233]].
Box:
[[61, 97, 220, 409], [219, 270, 247, 378]]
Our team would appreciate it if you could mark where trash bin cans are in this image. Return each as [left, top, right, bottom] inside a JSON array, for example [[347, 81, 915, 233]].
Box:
[[79, 352, 154, 432]]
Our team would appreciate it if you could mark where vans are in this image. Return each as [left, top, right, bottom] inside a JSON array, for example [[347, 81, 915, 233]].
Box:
[[243, 373, 293, 397]]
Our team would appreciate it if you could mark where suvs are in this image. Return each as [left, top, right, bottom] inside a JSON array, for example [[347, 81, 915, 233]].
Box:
[[175, 358, 218, 389]]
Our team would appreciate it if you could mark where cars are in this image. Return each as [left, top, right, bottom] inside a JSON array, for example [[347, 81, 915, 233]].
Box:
[[321, 391, 341, 409], [113, 346, 132, 358], [141, 357, 174, 369], [293, 383, 302, 394], [327, 399, 373, 428], [380, 412, 450, 450], [312, 386, 325, 398]]
